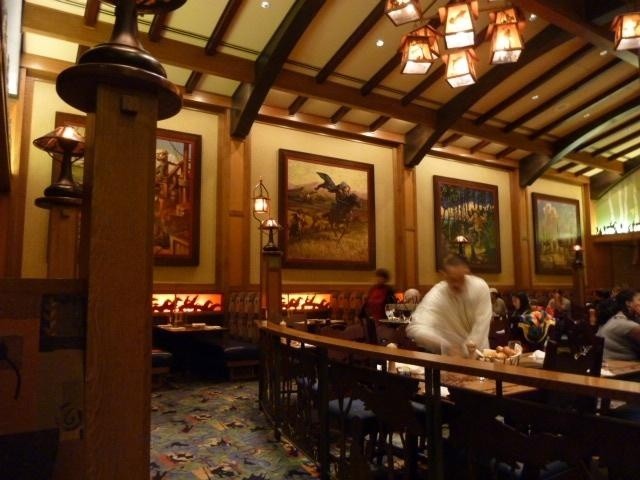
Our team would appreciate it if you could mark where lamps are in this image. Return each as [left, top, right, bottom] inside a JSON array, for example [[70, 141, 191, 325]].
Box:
[[258, 219, 284, 250], [452, 235, 469, 259], [33, 127, 90, 200], [253, 176, 270, 251], [610, 11, 640, 51], [383, 0, 526, 88]]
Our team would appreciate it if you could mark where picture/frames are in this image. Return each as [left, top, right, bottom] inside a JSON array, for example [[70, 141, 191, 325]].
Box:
[[46, 111, 201, 266], [532, 192, 581, 275], [433, 175, 501, 274], [278, 148, 376, 271]]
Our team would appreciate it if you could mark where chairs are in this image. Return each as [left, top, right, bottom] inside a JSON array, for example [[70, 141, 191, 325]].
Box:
[[186, 312, 262, 383]]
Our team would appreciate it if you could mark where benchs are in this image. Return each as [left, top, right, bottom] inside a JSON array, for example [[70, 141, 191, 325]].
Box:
[[153, 346, 173, 386]]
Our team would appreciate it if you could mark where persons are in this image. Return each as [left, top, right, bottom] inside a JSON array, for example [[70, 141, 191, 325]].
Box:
[[335, 180, 350, 203], [596, 280, 623, 324], [405, 253, 493, 359], [509, 291, 532, 338], [363, 269, 394, 343], [547, 287, 571, 320], [491, 288, 507, 316], [596, 287, 640, 380]]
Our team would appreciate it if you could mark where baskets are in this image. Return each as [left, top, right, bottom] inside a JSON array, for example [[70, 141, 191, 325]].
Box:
[[477, 355, 520, 366]]
